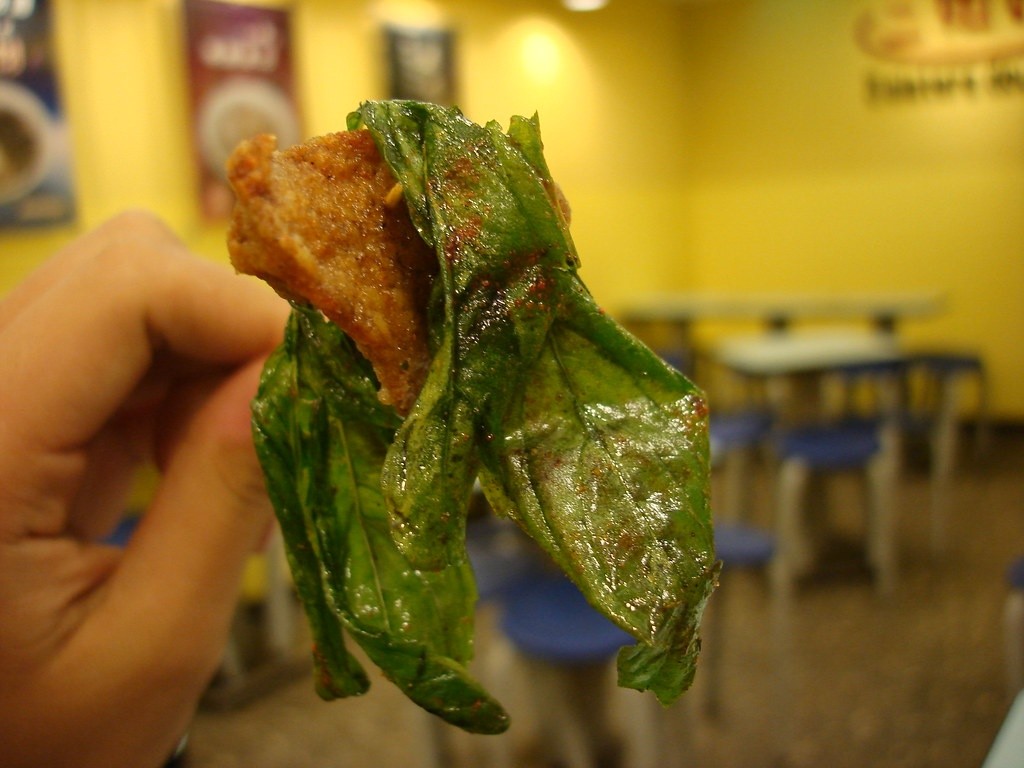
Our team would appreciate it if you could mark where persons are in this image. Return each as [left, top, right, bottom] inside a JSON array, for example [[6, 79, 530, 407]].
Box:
[[1, 206, 294, 768]]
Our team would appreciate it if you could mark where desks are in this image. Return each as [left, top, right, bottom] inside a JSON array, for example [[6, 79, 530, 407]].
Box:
[[613, 290, 989, 602]]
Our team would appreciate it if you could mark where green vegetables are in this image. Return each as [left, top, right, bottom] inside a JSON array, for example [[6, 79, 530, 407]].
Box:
[[248, 102, 725, 737]]
